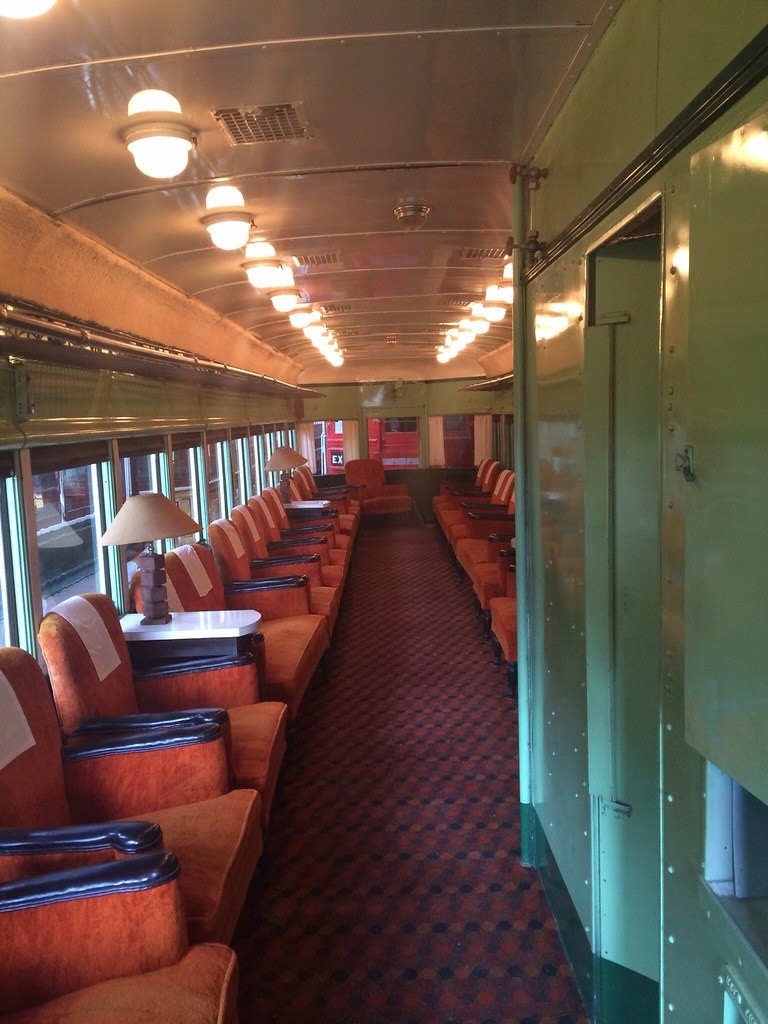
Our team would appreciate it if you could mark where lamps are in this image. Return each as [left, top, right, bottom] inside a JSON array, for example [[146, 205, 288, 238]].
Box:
[[264, 445, 308, 505], [97, 489, 203, 626], [0, 0, 517, 367]]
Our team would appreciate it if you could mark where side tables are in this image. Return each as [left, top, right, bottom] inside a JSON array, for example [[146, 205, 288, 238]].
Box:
[[116, 609, 262, 658]]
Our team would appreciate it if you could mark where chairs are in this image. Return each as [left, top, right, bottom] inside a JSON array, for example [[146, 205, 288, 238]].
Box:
[[133, 456, 517, 721], [0, 594, 288, 1024]]
